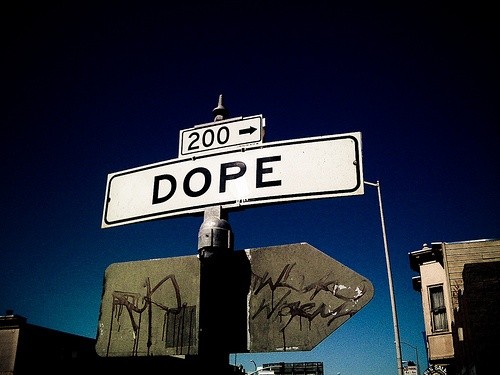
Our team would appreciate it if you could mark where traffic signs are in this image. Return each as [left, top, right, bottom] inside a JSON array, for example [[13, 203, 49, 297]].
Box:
[[100, 114, 365, 229]]
